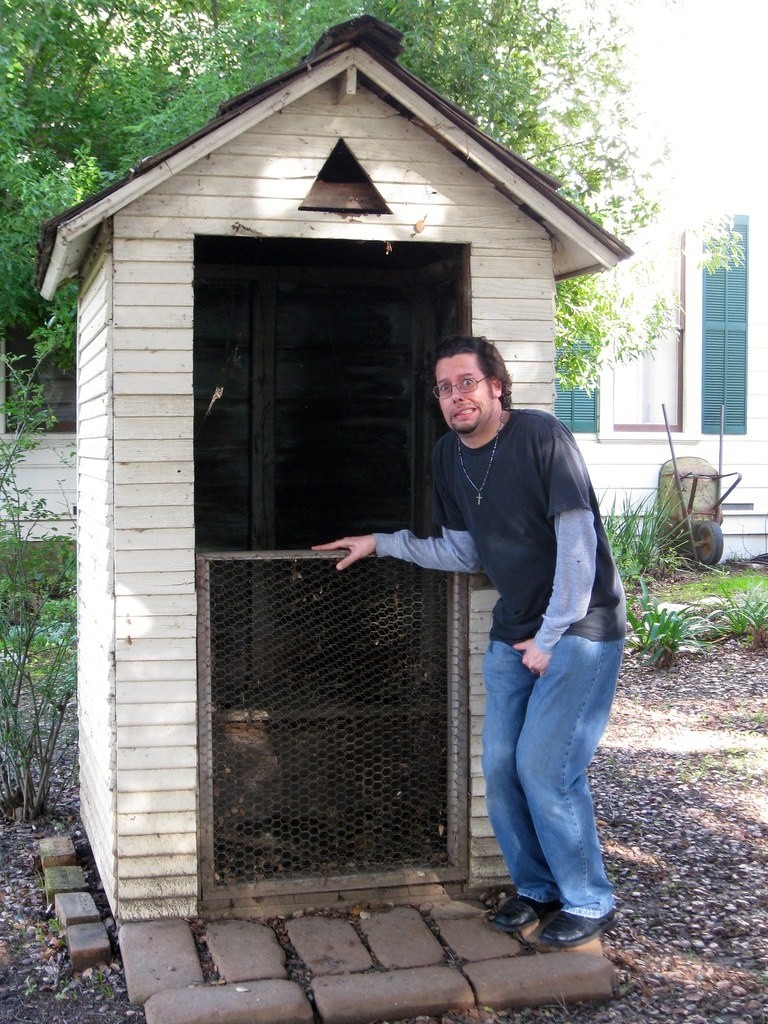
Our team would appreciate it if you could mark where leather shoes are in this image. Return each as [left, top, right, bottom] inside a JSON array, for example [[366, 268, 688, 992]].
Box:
[[539, 909, 618, 948], [492, 893, 565, 932]]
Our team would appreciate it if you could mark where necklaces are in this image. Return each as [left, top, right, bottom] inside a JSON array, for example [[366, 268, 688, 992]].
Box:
[[458, 412, 503, 504]]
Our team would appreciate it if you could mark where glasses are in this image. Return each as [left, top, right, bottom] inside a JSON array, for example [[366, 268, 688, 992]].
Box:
[[432, 377, 486, 398]]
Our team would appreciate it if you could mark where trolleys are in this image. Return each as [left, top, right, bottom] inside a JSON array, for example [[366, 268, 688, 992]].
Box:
[[655, 402, 742, 567]]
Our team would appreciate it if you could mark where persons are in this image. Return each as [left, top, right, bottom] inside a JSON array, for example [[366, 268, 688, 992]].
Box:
[[311, 337, 627, 948]]
